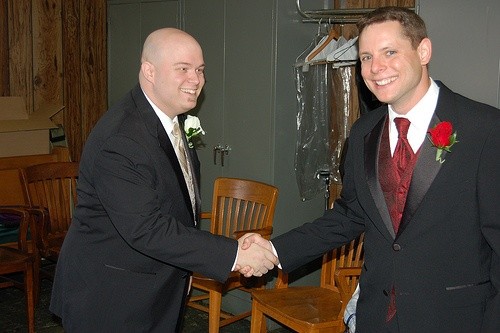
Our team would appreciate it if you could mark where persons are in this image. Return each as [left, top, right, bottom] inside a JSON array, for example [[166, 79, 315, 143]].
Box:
[[234, 6, 500, 333], [48, 27, 280, 333]]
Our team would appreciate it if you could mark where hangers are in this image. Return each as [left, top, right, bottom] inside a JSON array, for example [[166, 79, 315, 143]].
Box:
[[295, 17, 359, 68]]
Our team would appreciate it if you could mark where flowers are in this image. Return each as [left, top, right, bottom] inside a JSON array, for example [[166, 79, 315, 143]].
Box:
[[184, 115, 206, 149], [428, 121, 458, 163]]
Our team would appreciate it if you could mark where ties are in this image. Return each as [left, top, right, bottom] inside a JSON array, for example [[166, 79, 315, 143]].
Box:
[[172, 123, 191, 175], [393, 117, 413, 179]]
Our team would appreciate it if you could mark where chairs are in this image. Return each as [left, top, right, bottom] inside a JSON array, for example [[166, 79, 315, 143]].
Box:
[[250, 184, 365, 333], [20, 162, 79, 307], [186, 178, 278, 333], [0, 206, 35, 333]]
